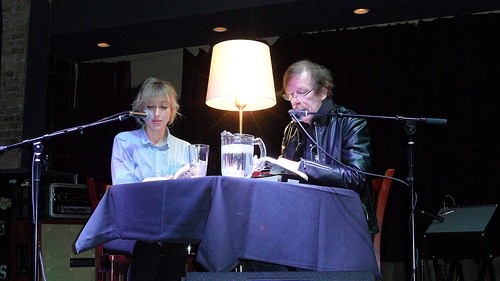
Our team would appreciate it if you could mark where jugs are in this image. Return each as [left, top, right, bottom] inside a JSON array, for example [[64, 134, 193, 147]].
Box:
[[220, 130, 266, 179]]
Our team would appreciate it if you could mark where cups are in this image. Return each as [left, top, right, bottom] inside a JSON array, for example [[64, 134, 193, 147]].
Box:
[[188, 143, 209, 178]]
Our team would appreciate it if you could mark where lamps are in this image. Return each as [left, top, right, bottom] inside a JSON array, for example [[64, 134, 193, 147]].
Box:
[[206, 39, 277, 134]]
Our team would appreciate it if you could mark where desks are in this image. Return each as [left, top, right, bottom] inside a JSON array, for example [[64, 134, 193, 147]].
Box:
[[75, 176, 381, 272], [7, 218, 97, 281]]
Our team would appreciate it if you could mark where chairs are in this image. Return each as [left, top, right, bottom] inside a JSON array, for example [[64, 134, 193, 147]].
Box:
[[184, 170, 395, 281], [85, 176, 195, 281]]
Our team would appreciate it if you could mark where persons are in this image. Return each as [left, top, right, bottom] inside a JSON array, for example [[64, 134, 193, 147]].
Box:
[[277, 61, 379, 243], [111, 77, 199, 281]]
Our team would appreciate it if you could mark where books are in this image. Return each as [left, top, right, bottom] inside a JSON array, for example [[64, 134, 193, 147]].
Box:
[[250, 154, 308, 183], [141, 163, 190, 182]]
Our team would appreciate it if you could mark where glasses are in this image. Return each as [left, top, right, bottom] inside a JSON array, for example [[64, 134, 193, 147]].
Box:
[[282, 87, 316, 101]]
